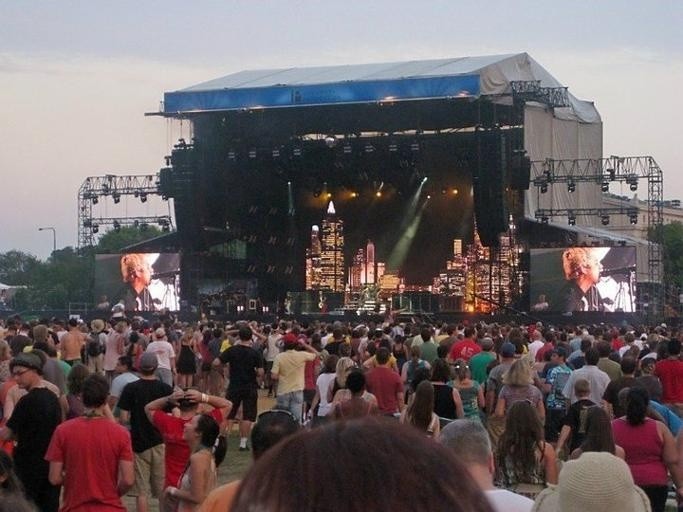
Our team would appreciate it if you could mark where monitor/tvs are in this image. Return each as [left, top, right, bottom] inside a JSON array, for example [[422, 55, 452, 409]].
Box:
[[529, 246, 637, 313], [92, 252, 183, 312]]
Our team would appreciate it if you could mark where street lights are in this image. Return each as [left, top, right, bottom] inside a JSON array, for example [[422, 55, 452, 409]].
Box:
[[39, 227, 56, 251]]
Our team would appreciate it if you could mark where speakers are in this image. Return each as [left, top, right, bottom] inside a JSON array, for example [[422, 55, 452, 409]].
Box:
[[510, 156, 531, 190], [160, 168, 175, 200]]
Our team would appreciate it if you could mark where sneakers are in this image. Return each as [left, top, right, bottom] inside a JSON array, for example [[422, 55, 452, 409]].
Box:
[[239, 444, 250, 452]]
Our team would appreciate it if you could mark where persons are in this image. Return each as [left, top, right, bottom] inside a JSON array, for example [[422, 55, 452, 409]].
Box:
[[551, 322, 683, 355], [318, 290, 330, 312], [270, 333, 319, 427], [264, 318, 284, 398], [11, 324, 32, 355], [161, 412, 227, 511], [208, 329, 223, 395], [364, 347, 406, 422], [449, 325, 482, 357], [486, 342, 546, 414], [325, 328, 344, 357], [94, 294, 111, 309], [342, 320, 439, 347], [636, 356, 663, 406], [311, 334, 329, 381], [535, 332, 555, 362], [563, 348, 612, 416], [103, 319, 126, 376], [30, 325, 58, 358], [480, 319, 543, 341], [128, 319, 149, 351], [556, 378, 600, 460], [212, 326, 264, 452], [0, 340, 16, 382], [602, 355, 649, 418], [612, 386, 683, 512], [363, 338, 399, 372], [321, 325, 334, 349], [297, 333, 321, 422], [437, 345, 451, 362], [88, 319, 108, 375], [111, 300, 128, 320], [541, 347, 573, 444], [447, 358, 486, 425], [44, 372, 136, 512], [440, 324, 462, 347], [557, 249, 603, 312], [108, 355, 140, 416], [230, 416, 497, 511], [639, 377, 683, 437], [327, 356, 359, 403], [528, 330, 545, 356], [112, 254, 161, 312], [567, 340, 592, 371], [61, 317, 86, 367], [416, 329, 439, 366], [404, 367, 431, 403], [47, 330, 62, 360], [487, 358, 545, 442], [327, 369, 379, 421], [175, 327, 199, 389], [125, 332, 143, 370], [116, 351, 180, 511], [284, 295, 293, 314], [571, 405, 625, 464], [33, 342, 71, 395], [336, 342, 352, 358], [4, 349, 64, 503], [310, 355, 339, 425], [330, 366, 377, 414], [145, 388, 232, 511], [654, 338, 683, 417], [392, 334, 409, 373], [197, 329, 213, 394], [593, 340, 623, 380], [532, 294, 547, 311], [146, 328, 178, 388], [428, 358, 464, 428], [402, 344, 432, 387], [492, 400, 558, 501], [225, 324, 267, 351], [198, 407, 304, 512], [0, 351, 62, 512], [467, 338, 497, 383]]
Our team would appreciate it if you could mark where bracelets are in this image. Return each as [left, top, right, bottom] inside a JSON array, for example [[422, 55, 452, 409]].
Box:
[[199, 392, 212, 405]]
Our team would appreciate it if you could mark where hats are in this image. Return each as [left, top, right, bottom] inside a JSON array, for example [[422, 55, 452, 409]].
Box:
[[139, 352, 158, 371], [9, 352, 44, 376], [155, 328, 166, 337], [90, 319, 105, 334], [501, 342, 517, 354], [283, 332, 297, 345]]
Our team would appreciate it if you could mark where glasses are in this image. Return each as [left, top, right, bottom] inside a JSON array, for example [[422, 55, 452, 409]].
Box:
[[13, 368, 32, 376], [258, 406, 299, 429]]
